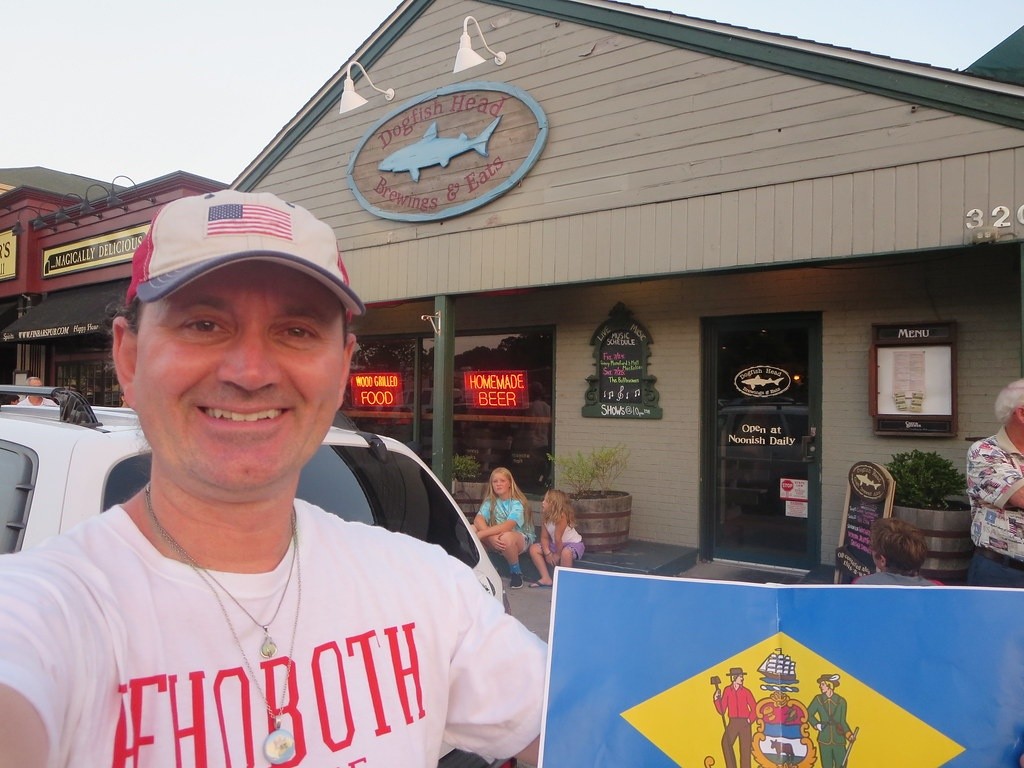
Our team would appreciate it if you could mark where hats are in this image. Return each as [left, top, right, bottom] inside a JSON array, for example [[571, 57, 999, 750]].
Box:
[[126, 190, 366, 325]]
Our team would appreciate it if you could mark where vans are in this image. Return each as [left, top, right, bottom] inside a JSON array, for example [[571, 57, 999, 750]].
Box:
[[373, 388, 465, 413]]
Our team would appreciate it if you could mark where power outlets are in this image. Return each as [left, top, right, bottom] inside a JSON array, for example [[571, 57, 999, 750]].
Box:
[[972, 226, 1000, 243]]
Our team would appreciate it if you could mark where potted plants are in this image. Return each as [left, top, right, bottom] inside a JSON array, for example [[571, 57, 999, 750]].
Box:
[[883, 448, 972, 580], [542, 443, 636, 553], [450, 452, 490, 523]]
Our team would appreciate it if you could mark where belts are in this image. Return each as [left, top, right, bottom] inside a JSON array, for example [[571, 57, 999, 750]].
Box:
[[975, 545, 1024, 570]]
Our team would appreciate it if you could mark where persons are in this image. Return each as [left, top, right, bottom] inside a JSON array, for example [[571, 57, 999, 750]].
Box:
[[851, 518, 944, 586], [966, 378, 1024, 588], [0, 189, 549, 768], [529, 489, 585, 589], [16, 375, 60, 406], [469, 468, 536, 589]]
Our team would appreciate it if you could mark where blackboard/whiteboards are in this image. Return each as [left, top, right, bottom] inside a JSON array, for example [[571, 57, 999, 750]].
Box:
[[599, 329, 644, 403], [834, 462, 896, 577]]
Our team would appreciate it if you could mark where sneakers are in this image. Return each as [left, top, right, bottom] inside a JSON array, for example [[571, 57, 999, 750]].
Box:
[[510, 574, 523, 589]]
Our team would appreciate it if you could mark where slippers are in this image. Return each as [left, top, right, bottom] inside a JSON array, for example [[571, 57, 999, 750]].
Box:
[[528, 578, 556, 588]]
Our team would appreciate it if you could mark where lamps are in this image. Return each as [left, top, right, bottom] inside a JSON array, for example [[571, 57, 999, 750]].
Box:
[[338, 60, 395, 113], [79, 184, 128, 216], [106, 175, 156, 213], [56, 191, 104, 224], [33, 201, 80, 232], [12, 206, 55, 236], [453, 13, 505, 73]]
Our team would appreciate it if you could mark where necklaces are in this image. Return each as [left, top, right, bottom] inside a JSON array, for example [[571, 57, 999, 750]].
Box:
[[145, 480, 297, 657], [145, 484, 300, 764]]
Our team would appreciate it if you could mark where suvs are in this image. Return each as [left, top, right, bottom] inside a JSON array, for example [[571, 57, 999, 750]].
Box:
[[715, 396, 809, 490], [0, 384, 511, 619]]
